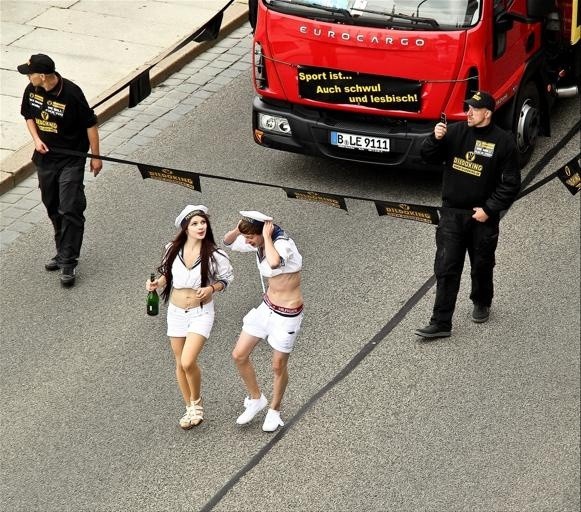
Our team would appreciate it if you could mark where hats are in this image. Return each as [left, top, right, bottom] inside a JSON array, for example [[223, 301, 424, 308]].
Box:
[[239, 211, 273, 223], [175, 204, 209, 228], [17, 54, 55, 74], [463, 90, 495, 112]]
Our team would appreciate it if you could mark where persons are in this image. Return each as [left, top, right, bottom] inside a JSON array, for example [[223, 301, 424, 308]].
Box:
[[146, 204, 235, 431], [413, 92, 522, 339], [223, 209, 308, 433], [17, 54, 102, 287]]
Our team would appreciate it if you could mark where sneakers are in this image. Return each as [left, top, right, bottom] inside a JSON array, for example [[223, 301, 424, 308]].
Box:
[[415, 324, 451, 338], [237, 393, 267, 424], [45, 258, 61, 270], [180, 396, 204, 430], [472, 303, 490, 322], [262, 408, 284, 431], [61, 267, 75, 283]]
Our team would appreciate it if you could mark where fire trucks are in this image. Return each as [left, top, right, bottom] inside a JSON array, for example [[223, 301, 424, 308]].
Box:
[[248, 0, 579, 187]]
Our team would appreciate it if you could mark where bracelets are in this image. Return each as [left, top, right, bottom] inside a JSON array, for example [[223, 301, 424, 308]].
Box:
[[210, 285, 215, 294]]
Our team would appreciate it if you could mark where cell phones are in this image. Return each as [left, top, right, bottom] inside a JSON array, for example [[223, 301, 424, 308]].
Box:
[[441, 113, 447, 124]]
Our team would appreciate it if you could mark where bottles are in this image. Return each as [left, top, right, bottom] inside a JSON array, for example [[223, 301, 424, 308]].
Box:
[[146, 272, 160, 317]]
